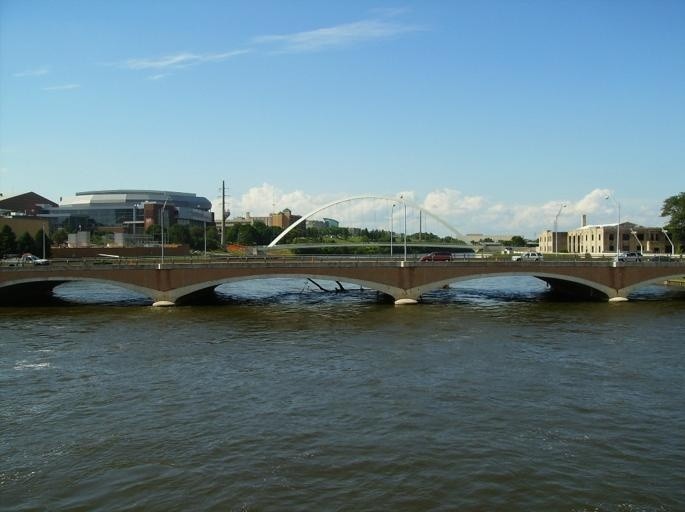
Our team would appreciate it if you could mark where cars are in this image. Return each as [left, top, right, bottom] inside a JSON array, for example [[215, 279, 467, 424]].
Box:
[[650, 256, 678, 263]]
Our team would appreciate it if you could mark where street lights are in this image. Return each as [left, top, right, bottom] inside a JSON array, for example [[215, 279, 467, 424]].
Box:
[[554, 202, 568, 256], [603, 191, 621, 258], [390, 193, 408, 262]]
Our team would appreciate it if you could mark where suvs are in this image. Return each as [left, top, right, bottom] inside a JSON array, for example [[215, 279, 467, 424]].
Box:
[[614, 252, 643, 262], [511, 252, 543, 264]]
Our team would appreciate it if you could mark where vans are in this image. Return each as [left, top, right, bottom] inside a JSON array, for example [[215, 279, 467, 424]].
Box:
[[419, 251, 453, 262]]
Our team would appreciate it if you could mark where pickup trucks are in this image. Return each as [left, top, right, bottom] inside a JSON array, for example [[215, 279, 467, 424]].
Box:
[[5, 253, 49, 267]]
